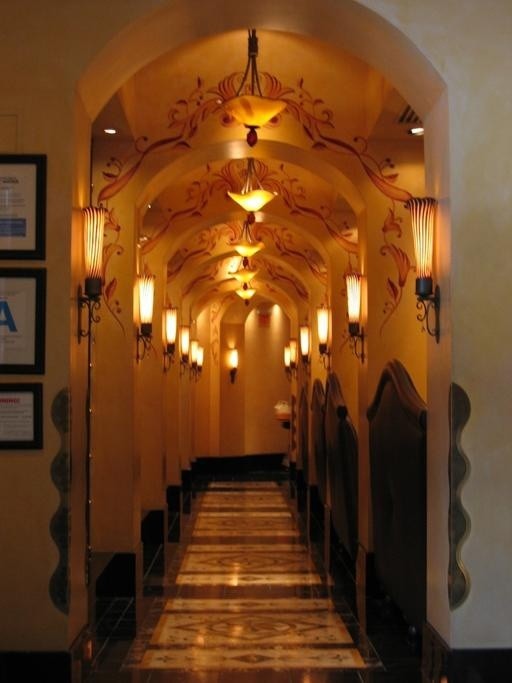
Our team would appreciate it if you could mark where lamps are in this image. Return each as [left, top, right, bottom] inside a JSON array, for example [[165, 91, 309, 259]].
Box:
[[165, 305, 204, 384], [403, 196, 442, 344], [342, 271, 367, 365], [282, 301, 332, 383], [138, 270, 157, 362], [222, 27, 288, 307], [77, 203, 113, 352]]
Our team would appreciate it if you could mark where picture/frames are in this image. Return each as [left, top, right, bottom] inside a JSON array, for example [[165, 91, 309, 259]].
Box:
[[0, 152, 47, 453]]
